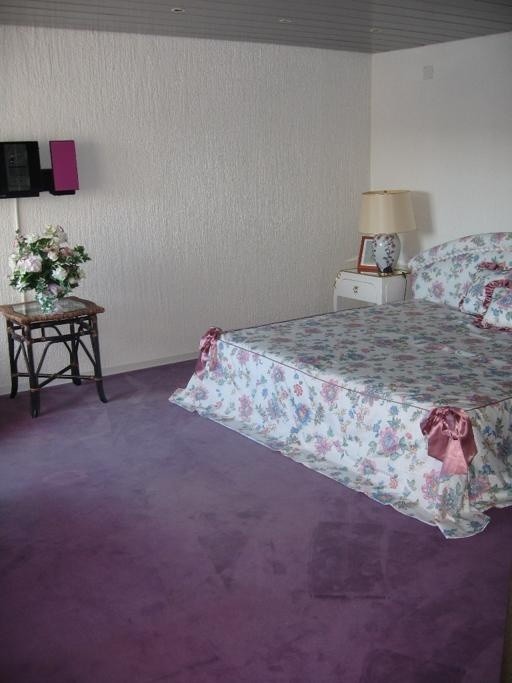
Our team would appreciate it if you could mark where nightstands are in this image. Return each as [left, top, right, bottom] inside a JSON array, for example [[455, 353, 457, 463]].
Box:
[[332, 268, 413, 312]]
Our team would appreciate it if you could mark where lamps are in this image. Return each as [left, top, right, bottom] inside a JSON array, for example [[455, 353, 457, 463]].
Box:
[[358, 189, 417, 277]]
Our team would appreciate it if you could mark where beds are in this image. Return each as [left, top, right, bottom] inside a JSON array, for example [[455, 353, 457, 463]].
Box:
[[168, 231, 512, 541]]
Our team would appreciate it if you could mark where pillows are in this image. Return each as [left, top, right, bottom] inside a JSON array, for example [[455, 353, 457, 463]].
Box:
[[469, 279, 512, 334], [458, 261, 512, 317]]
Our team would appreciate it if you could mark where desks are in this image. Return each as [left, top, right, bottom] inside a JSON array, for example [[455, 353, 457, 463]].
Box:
[[0, 296, 108, 419]]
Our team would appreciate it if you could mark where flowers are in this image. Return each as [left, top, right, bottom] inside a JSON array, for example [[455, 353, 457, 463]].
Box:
[[5, 224, 92, 314]]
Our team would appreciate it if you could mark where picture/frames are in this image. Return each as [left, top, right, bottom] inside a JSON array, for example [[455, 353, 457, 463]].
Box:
[[357, 236, 379, 274]]
